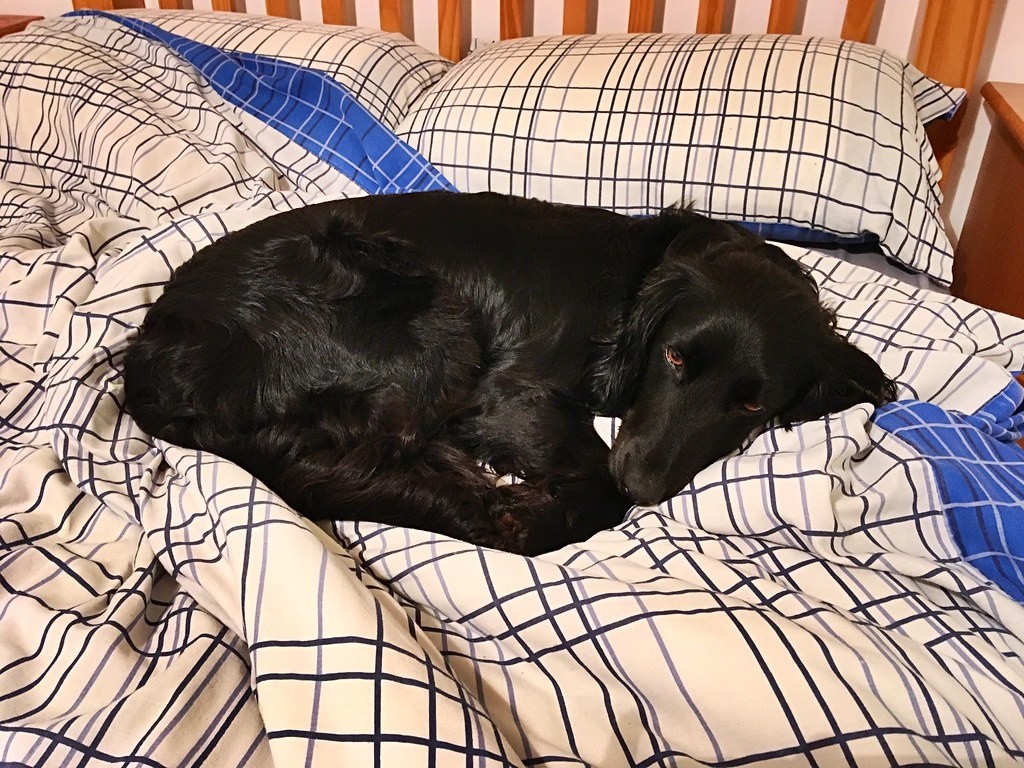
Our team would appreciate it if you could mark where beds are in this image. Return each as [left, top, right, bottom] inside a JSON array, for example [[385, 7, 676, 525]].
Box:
[[0, 0, 1024, 768]]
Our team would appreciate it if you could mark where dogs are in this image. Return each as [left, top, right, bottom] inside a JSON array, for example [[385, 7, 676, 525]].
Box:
[[123, 189, 901, 558]]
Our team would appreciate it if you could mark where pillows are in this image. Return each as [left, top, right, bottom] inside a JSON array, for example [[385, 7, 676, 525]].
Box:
[[106, 8, 457, 129], [393, 33, 969, 287]]
